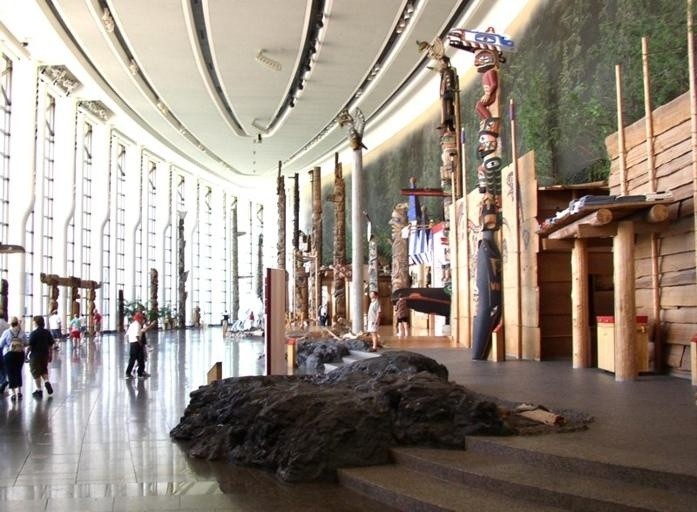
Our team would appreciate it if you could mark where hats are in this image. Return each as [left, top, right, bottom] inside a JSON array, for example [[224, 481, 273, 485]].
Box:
[[133, 312, 144, 321], [11, 316, 18, 325]]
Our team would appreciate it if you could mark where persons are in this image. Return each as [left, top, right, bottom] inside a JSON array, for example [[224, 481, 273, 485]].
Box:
[[367, 292, 382, 352], [123, 311, 153, 380], [424, 49, 506, 362], [317, 305, 330, 326], [394, 294, 411, 338], [248, 311, 255, 331], [0, 308, 103, 401], [221, 310, 231, 338]]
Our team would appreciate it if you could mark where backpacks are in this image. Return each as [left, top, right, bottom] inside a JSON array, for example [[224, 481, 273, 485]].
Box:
[[8, 327, 23, 353]]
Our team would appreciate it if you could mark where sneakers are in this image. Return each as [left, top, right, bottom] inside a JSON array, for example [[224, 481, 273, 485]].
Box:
[[45, 381, 53, 395], [11, 393, 23, 400], [368, 346, 377, 351], [32, 390, 43, 397], [0, 380, 8, 394], [125, 369, 152, 378]]
